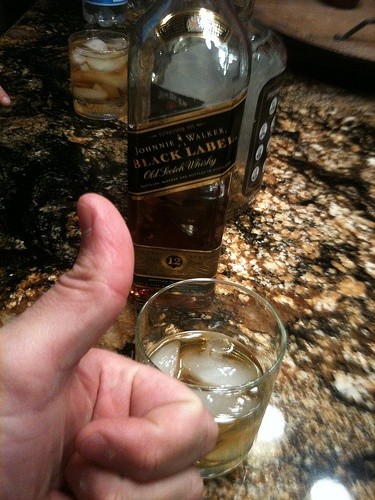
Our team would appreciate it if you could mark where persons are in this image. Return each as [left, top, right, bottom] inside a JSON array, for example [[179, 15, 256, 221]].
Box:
[[0, 194, 218, 500]]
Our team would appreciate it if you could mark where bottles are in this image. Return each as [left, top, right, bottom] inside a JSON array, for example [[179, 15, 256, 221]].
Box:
[[122, 0, 252, 308], [82, 0, 128, 27], [225, 1, 288, 220]]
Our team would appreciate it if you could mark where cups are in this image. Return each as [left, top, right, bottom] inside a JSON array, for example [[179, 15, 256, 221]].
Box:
[[134, 278, 287, 478], [68, 29, 132, 120]]
